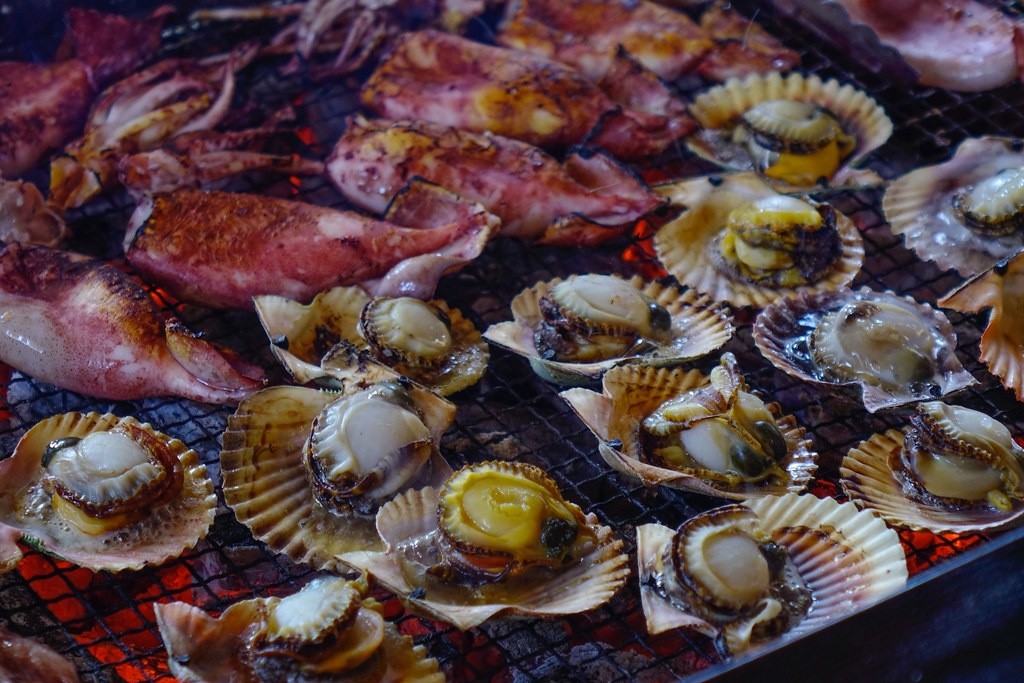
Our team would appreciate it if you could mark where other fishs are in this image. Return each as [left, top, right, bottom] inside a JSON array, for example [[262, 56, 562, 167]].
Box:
[[757, 0, 1024, 93], [1, 0, 801, 408]]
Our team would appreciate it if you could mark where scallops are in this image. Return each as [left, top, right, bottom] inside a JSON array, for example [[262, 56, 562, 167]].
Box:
[[479, 71, 1024, 666], [332, 459, 633, 635], [0, 411, 219, 575], [153, 567, 449, 683], [251, 286, 492, 399], [219, 336, 460, 578]]
